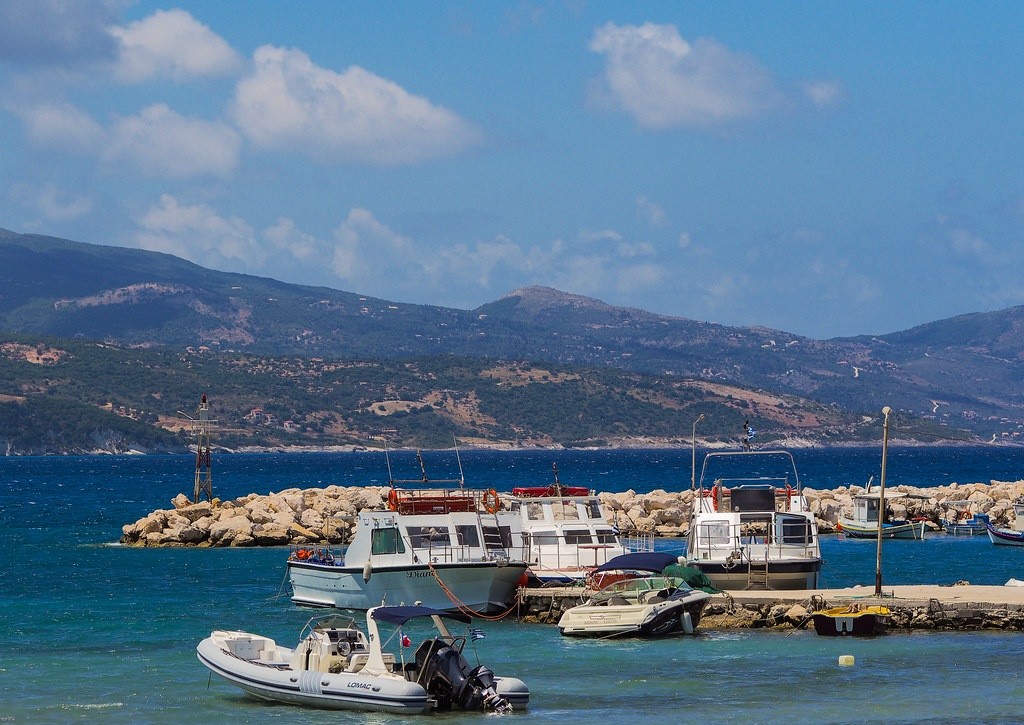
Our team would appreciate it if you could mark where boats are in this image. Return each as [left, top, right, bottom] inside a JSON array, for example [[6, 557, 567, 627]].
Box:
[[943, 500, 992, 536], [286, 431, 655, 618], [557, 578, 711, 639], [196, 592, 531, 717], [808, 591, 894, 637], [683, 420, 823, 590], [837, 475, 932, 540], [987, 501, 1024, 545]]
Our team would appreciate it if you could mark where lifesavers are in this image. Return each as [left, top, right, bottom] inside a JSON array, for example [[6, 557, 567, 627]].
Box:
[[483, 489, 500, 514], [786, 484, 791, 510], [712, 486, 719, 511], [307, 549, 323, 559], [962, 510, 973, 520], [388, 490, 400, 510]]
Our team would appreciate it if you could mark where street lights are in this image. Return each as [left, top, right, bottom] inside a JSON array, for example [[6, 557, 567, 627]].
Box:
[[688, 414, 704, 527], [874, 406, 893, 593]]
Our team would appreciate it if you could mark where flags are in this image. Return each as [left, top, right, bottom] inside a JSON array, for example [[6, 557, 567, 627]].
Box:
[[401, 630, 411, 649], [470, 627, 486, 642]]
